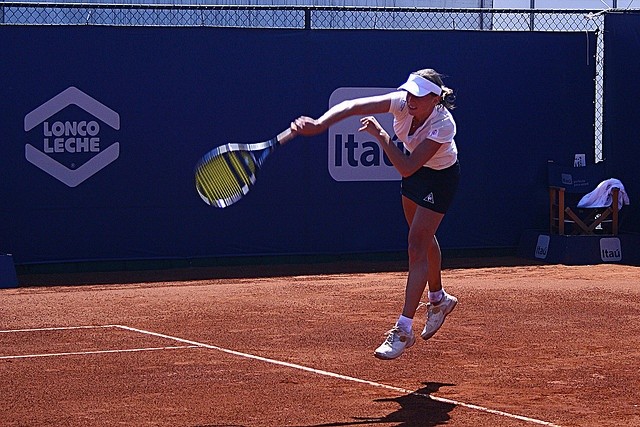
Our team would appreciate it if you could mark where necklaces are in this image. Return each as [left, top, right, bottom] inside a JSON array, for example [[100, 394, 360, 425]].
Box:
[[413, 125, 415, 127]]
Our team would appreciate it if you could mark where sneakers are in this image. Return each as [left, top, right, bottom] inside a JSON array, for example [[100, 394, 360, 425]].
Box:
[[420, 290, 458, 340], [373, 325, 416, 360]]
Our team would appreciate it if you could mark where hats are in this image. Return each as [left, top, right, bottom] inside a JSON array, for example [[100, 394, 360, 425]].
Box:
[[396, 74, 443, 96]]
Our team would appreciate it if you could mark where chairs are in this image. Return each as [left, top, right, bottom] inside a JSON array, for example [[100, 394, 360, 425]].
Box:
[[549, 158, 619, 236]]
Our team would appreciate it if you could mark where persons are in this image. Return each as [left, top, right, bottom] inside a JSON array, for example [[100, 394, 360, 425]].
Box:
[[290, 67, 461, 359]]
